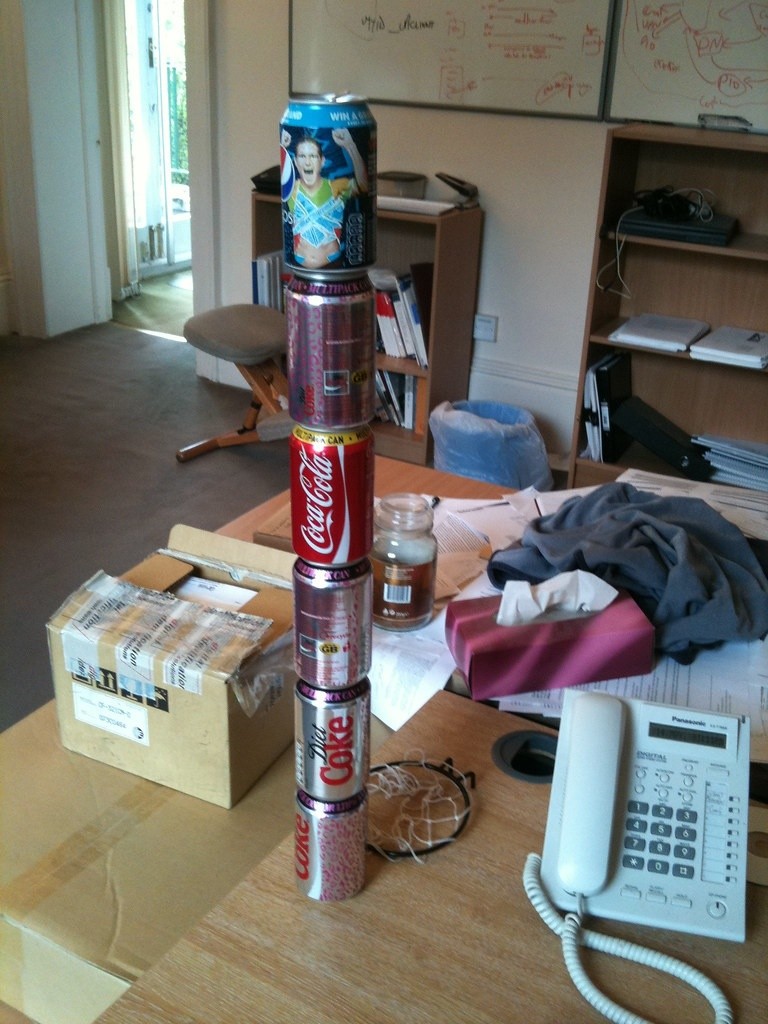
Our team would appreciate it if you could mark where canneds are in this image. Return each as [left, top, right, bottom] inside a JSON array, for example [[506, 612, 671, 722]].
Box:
[[293, 677, 371, 803], [294, 789, 369, 902], [284, 269, 376, 429], [277, 89, 377, 273], [291, 556, 373, 690], [289, 422, 375, 565]]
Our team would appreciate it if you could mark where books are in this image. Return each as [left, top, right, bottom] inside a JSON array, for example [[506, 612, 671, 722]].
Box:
[[581, 297, 768, 495], [242, 252, 429, 433]]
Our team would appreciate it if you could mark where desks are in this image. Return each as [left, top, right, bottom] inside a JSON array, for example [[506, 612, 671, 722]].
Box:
[[0, 450, 768, 1024]]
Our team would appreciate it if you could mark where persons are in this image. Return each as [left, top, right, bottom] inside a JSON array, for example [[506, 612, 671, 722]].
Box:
[[281, 128, 368, 272]]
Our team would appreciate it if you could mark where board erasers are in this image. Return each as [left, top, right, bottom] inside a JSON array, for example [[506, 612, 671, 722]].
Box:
[[697, 113, 751, 130]]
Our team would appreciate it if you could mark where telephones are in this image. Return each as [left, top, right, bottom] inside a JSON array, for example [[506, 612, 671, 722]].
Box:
[[539, 686, 751, 944]]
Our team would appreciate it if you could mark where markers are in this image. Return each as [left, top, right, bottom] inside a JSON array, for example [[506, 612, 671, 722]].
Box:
[[624, 118, 680, 127]]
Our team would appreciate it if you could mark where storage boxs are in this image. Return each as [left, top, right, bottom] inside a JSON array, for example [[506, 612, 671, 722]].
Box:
[[46, 523, 302, 807]]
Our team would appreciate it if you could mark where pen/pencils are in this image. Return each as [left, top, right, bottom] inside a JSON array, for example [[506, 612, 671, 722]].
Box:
[[431, 495, 441, 507]]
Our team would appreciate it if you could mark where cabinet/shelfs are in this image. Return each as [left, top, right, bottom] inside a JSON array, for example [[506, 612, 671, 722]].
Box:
[[250, 169, 485, 467], [565, 124, 767, 540]]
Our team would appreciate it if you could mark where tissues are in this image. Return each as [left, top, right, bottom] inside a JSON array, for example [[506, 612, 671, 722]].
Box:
[[445, 569, 655, 700]]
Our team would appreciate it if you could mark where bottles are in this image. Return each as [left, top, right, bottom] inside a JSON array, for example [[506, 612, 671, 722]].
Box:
[[372, 492, 438, 631]]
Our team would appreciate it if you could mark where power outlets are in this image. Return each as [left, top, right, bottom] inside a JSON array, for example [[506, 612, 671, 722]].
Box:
[[473, 314, 497, 342]]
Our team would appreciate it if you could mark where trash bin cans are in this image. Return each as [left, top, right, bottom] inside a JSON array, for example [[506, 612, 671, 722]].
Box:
[[428, 398, 554, 493]]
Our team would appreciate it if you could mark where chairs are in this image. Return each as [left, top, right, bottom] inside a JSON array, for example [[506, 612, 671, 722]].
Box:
[[176, 303, 293, 463]]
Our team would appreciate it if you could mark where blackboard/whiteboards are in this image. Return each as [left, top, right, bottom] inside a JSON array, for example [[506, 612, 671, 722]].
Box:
[[289, 1, 768, 135]]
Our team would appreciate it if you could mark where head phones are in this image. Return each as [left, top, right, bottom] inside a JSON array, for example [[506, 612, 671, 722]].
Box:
[[638, 190, 710, 222]]
